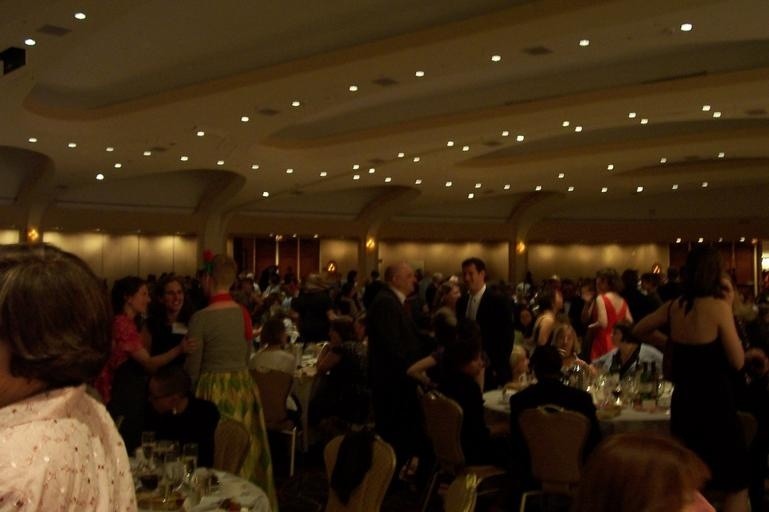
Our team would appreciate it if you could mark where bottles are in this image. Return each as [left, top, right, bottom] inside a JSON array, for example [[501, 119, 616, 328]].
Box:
[[651, 359, 658, 398], [639, 362, 651, 405]]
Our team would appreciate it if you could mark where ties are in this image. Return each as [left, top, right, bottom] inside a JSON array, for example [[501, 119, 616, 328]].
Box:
[[468, 295, 475, 319]]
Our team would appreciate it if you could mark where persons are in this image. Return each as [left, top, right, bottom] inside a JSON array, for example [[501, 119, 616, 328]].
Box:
[[363, 271, 384, 310], [587, 270, 633, 363], [407, 256, 515, 465], [294, 319, 367, 443], [530, 281, 564, 346], [549, 325, 597, 377], [433, 282, 462, 345], [251, 320, 296, 431], [618, 268, 647, 342], [144, 275, 211, 364], [93, 275, 200, 444], [592, 323, 665, 380], [0, 243, 138, 512], [367, 260, 436, 494], [251, 293, 283, 330], [182, 256, 278, 512], [516, 272, 532, 298], [430, 336, 491, 488], [756, 270, 769, 323], [346, 320, 369, 386], [632, 244, 751, 512], [263, 279, 280, 297], [402, 268, 432, 306], [516, 305, 534, 346], [342, 271, 368, 315], [298, 271, 353, 340], [569, 282, 592, 338], [508, 344, 601, 502], [240, 279, 265, 306], [427, 272, 444, 308], [641, 271, 663, 308], [287, 296, 306, 348]]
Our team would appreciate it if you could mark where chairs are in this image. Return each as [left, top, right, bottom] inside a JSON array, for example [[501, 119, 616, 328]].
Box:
[[324, 433, 396, 512], [419, 387, 508, 512], [250, 366, 309, 477], [520, 405, 591, 512], [214, 416, 250, 474], [737, 410, 756, 447]]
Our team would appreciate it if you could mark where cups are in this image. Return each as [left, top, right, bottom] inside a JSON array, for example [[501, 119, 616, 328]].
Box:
[[184, 456, 197, 491], [592, 378, 610, 418], [642, 399, 656, 413], [141, 431, 153, 467], [155, 441, 168, 469], [659, 395, 671, 407], [140, 469, 159, 509], [621, 376, 639, 413], [161, 461, 180, 503]]
